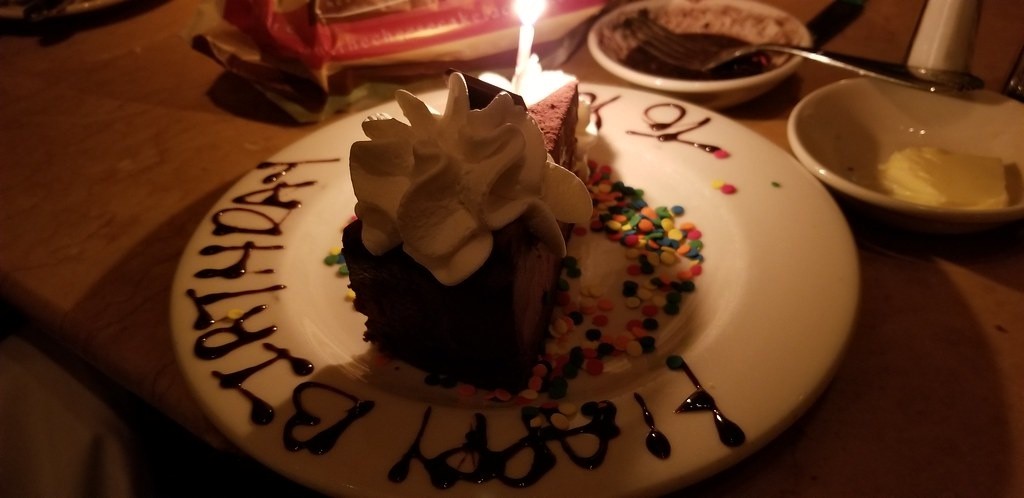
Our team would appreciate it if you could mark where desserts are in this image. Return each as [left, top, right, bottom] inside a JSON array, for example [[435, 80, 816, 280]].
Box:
[[337, 55, 594, 391]]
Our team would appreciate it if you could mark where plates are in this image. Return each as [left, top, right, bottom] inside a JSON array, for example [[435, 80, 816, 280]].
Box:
[[169, 79, 863, 497], [588, 0, 813, 109], [786, 74, 1024, 238]]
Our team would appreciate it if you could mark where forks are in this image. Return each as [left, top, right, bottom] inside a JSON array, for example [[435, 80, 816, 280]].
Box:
[[626, 16, 984, 93]]
